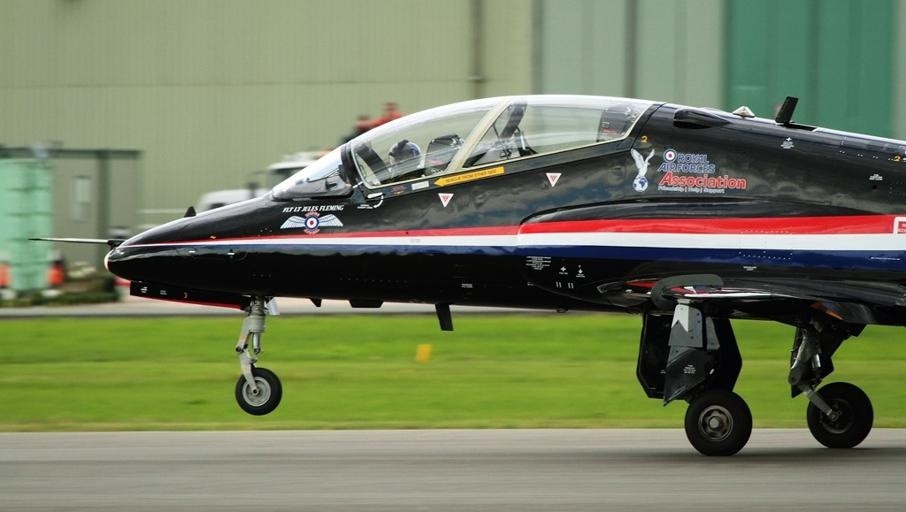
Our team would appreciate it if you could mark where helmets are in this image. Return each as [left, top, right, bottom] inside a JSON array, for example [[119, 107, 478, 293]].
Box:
[[387, 139, 421, 182], [597, 105, 642, 142], [424, 134, 464, 176]]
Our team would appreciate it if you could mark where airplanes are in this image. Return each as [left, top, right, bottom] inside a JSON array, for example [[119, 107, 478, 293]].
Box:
[[30, 90, 905, 456]]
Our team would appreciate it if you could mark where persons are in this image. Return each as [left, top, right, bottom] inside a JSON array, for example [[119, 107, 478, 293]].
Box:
[[342, 114, 373, 144], [387, 139, 425, 185], [357, 101, 404, 129]]
